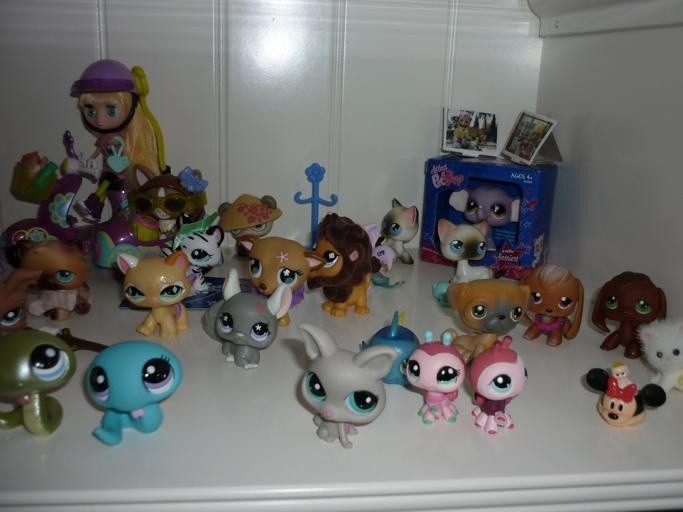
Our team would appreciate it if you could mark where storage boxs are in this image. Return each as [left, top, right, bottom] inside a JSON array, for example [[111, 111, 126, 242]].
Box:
[[419, 153, 558, 272]]
